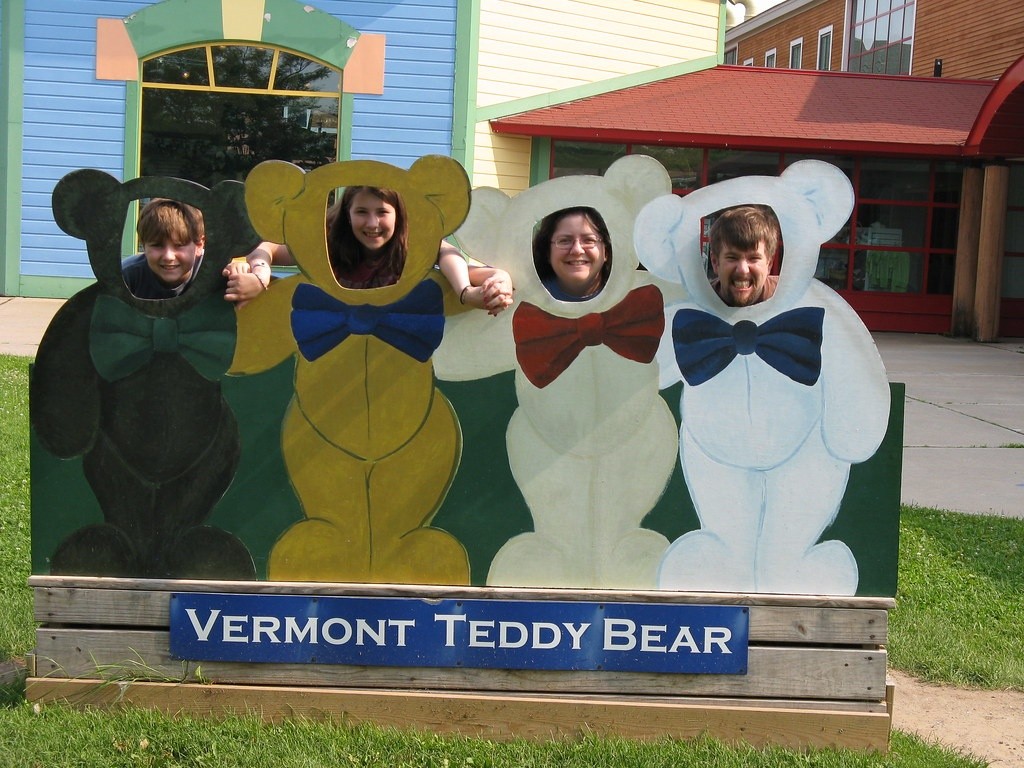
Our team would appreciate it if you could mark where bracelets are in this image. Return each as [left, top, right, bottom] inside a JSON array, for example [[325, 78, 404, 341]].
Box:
[[252, 263, 271, 269], [253, 272, 269, 291], [460, 285, 468, 305]]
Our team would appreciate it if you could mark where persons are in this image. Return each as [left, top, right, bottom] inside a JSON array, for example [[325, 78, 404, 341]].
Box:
[[225, 186, 513, 309], [709, 207, 780, 307], [468, 206, 612, 316], [122, 198, 280, 300]]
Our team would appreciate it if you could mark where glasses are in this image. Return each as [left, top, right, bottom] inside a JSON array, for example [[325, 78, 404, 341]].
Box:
[[550, 236, 604, 249]]
